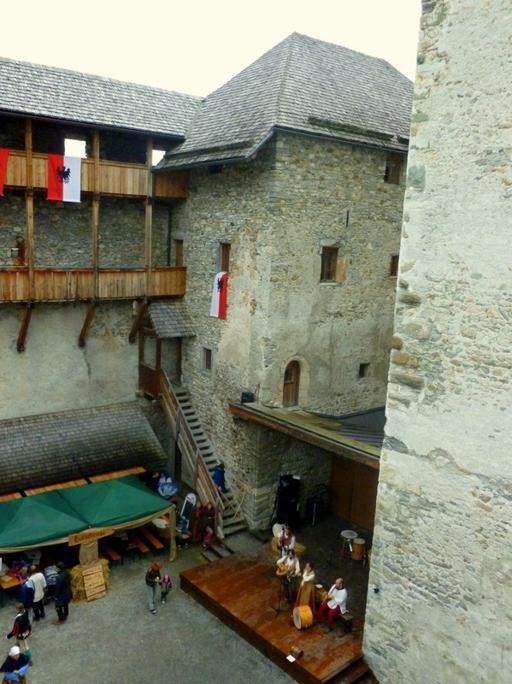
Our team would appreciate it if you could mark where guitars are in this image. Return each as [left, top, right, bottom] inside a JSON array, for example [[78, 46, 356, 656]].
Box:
[[276, 540, 289, 551]]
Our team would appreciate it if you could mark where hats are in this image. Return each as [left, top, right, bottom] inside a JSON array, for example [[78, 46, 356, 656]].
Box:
[[8, 645, 20, 656], [151, 560, 161, 571]]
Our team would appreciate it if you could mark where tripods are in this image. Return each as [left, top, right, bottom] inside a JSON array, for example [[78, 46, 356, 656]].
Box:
[[272, 552, 284, 618]]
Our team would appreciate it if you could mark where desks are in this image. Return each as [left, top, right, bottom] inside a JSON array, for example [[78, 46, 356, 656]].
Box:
[[0, 572, 21, 609]]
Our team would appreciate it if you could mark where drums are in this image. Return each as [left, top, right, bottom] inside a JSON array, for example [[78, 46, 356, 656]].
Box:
[[351, 539, 366, 561], [293, 605, 314, 630]]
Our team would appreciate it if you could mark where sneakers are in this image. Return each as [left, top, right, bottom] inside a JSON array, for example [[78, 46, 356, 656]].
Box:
[[151, 599, 166, 615]]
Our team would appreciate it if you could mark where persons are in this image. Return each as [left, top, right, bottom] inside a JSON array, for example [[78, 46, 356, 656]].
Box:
[[162, 575, 172, 604], [277, 549, 300, 602], [279, 527, 296, 557], [0, 646, 30, 684], [211, 463, 230, 493], [317, 577, 348, 630], [299, 562, 316, 608], [18, 565, 47, 621], [145, 561, 162, 614], [4, 603, 32, 666], [53, 562, 71, 624]]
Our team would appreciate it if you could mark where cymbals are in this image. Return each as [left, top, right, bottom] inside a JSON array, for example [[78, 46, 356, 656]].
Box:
[[276, 566, 288, 576]]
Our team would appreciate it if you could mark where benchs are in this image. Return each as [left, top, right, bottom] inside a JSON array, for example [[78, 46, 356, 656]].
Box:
[[101, 530, 166, 566]]
